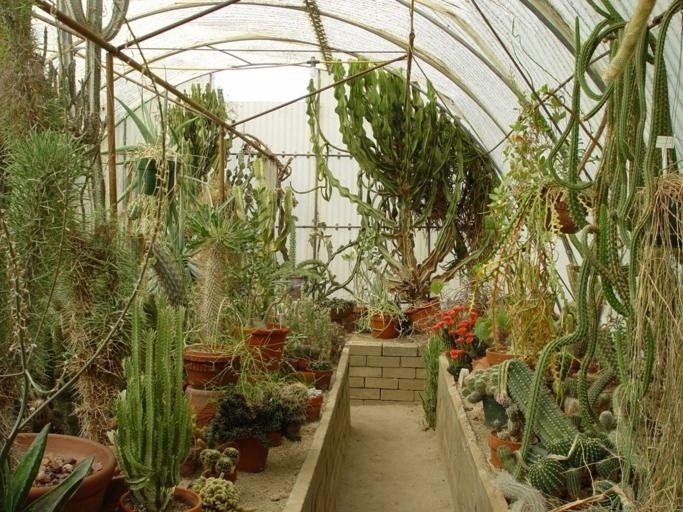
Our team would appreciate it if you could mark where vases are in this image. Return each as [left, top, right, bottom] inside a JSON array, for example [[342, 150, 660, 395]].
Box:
[[185, 386, 234, 430], [482, 394, 505, 426], [298, 358, 307, 370], [8, 422, 117, 512]]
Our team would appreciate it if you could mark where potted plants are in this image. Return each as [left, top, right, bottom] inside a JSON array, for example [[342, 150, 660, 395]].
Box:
[[204, 392, 302, 474], [200, 447, 240, 486], [302, 58, 464, 332], [306, 361, 333, 391], [304, 383, 322, 422], [351, 237, 408, 339], [173, 185, 291, 389], [105, 287, 202, 512], [488, 404, 538, 471], [97, 64, 224, 215], [318, 293, 357, 324], [283, 334, 298, 371], [467, 83, 586, 403]]
